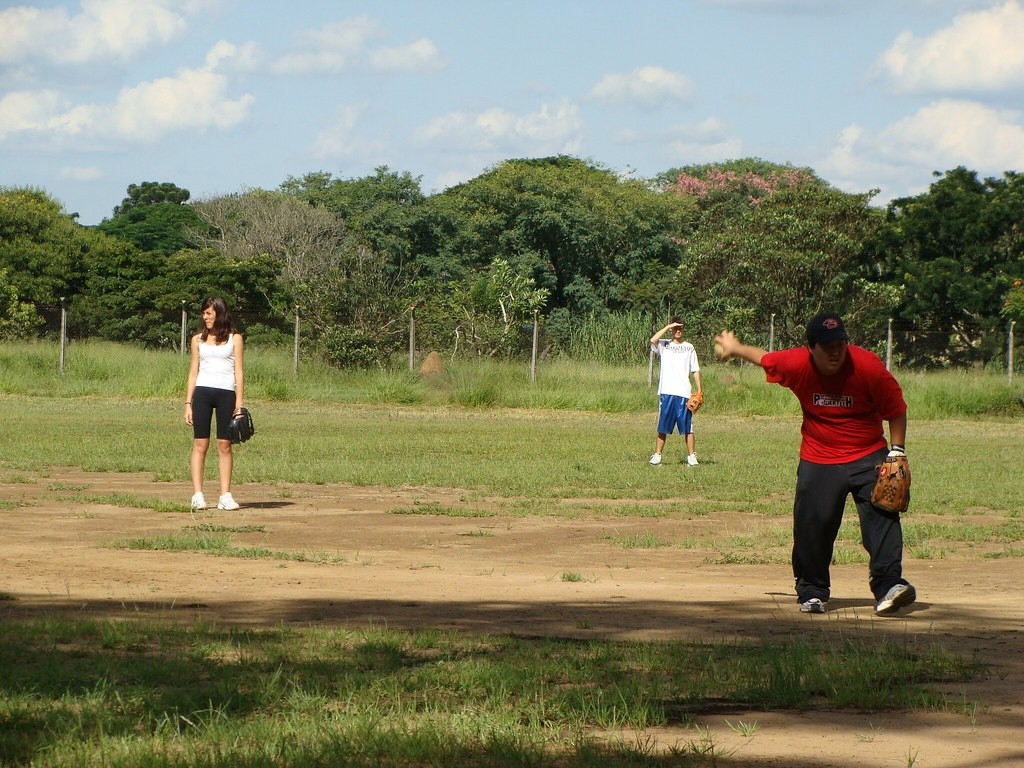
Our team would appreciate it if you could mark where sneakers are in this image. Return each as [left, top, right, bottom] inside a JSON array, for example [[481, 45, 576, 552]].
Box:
[[649, 453, 662, 466], [800, 598, 825, 613], [873, 583, 916, 615], [218, 492, 239, 510], [191, 492, 207, 510], [687, 452, 699, 466]]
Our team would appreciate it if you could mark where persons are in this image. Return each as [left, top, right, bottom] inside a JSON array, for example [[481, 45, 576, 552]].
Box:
[[649, 316, 703, 465], [715, 313, 916, 615], [184, 296, 244, 511]]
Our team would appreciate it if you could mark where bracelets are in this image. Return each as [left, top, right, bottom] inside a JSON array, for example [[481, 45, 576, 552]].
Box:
[[185, 402, 191, 404]]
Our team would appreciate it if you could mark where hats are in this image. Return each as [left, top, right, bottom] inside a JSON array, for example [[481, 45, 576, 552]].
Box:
[[805, 312, 850, 343]]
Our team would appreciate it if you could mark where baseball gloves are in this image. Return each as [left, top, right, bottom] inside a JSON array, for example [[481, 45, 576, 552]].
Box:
[[227, 409, 254, 444], [869, 455, 910, 512], [685, 391, 702, 413]]
